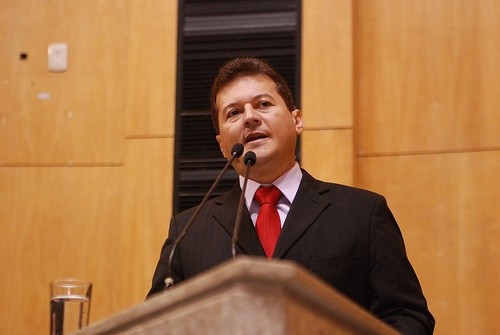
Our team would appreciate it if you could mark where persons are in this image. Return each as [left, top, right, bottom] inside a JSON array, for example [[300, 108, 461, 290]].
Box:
[[144, 57, 435, 335]]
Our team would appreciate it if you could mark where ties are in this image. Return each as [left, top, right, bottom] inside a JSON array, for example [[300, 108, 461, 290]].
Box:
[[252, 185, 283, 261]]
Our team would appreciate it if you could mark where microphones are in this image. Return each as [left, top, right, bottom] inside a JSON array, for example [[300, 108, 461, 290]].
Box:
[[163, 142, 245, 289], [230, 150, 257, 257]]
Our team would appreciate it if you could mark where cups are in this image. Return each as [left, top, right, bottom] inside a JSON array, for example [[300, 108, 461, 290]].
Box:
[[49, 280, 93, 335]]
